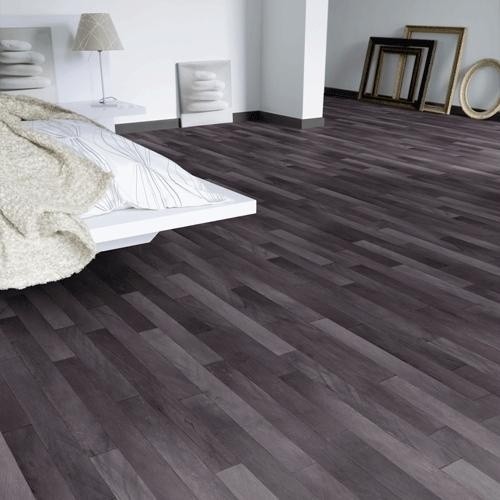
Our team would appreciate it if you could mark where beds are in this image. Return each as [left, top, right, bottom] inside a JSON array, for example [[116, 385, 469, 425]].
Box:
[[0, 27, 257, 292]]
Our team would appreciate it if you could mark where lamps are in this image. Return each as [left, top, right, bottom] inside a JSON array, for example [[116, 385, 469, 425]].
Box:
[[72, 12, 124, 108]]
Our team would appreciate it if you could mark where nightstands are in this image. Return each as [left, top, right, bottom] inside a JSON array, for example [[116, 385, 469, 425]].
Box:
[[54, 97, 146, 134]]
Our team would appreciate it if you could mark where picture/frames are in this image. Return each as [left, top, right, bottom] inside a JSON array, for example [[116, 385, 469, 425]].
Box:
[[356, 26, 500, 119]]
